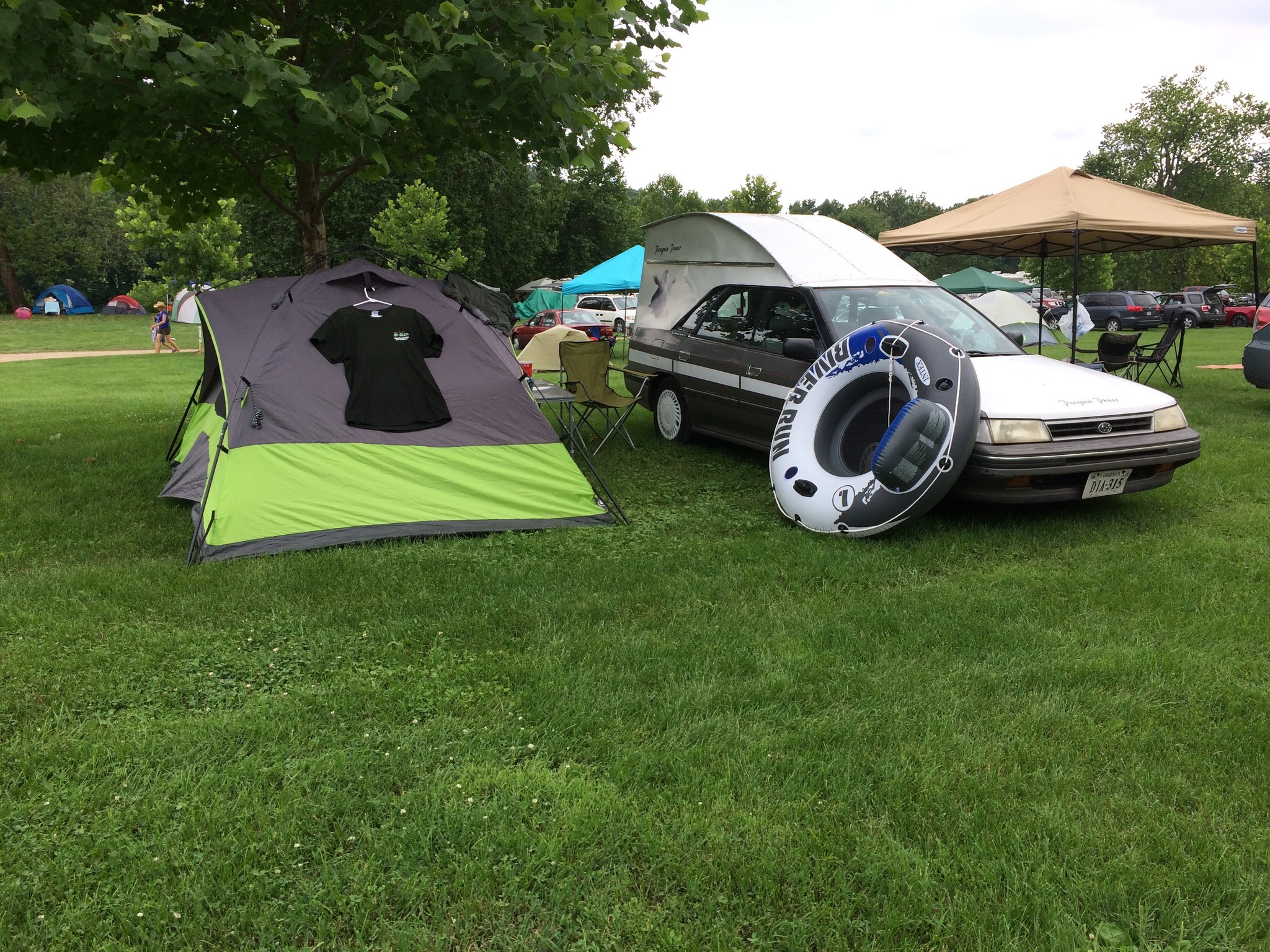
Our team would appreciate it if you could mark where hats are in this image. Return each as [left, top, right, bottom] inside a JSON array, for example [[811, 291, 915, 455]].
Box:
[[154, 301, 167, 308]]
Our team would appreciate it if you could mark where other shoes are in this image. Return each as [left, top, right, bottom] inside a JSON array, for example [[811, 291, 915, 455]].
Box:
[[171, 349, 177, 353]]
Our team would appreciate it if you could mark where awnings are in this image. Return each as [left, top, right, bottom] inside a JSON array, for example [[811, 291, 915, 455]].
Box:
[[874, 162, 1263, 368], [561, 245, 656, 294]]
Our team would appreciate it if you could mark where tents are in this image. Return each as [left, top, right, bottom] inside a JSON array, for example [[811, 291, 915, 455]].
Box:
[[31, 283, 95, 315], [171, 282, 219, 324], [100, 293, 148, 315], [949, 288, 1063, 353], [155, 256, 630, 555]]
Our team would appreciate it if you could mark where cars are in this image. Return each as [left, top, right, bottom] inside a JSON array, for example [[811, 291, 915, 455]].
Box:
[[1010, 288, 1066, 318], [623, 284, 1201, 507], [1216, 292, 1270, 390], [510, 309, 613, 350]]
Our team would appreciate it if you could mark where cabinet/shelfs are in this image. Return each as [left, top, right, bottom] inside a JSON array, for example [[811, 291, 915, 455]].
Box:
[[350, 285, 396, 312]]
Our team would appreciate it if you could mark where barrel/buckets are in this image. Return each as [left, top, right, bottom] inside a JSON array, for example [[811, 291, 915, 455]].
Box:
[[525, 378, 534, 391], [519, 361, 532, 377]]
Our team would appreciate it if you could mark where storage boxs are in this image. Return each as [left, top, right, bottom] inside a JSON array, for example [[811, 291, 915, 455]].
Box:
[[518, 360, 533, 377]]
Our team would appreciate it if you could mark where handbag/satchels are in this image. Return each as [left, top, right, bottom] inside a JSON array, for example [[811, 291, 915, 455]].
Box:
[[1058, 295, 1095, 343]]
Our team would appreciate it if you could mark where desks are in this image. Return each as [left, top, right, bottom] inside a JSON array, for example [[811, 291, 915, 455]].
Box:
[[526, 378, 576, 461], [43, 301, 60, 317]]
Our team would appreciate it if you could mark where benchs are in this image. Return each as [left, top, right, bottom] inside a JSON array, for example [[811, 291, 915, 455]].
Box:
[[716, 318, 747, 337]]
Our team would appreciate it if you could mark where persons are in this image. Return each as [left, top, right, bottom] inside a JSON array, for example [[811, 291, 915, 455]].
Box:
[[150, 301, 180, 354]]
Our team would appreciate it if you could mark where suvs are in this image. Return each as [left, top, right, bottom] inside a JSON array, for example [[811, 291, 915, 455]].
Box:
[[1043, 291, 1182, 332], [1144, 284, 1256, 329], [575, 293, 639, 333]]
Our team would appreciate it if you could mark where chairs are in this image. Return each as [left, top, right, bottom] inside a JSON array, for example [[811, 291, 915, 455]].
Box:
[[1092, 312, 1192, 389], [591, 327, 618, 359], [857, 306, 897, 326], [558, 340, 659, 455]]
